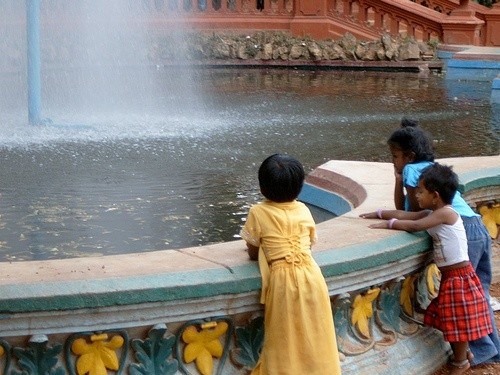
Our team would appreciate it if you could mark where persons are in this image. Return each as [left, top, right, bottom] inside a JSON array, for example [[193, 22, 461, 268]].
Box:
[[359, 163, 494, 375], [386, 117, 500, 367], [238, 154, 342, 375]]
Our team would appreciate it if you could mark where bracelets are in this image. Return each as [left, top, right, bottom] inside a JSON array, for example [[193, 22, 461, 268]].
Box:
[[389, 218, 397, 229], [377, 209, 383, 219]]
